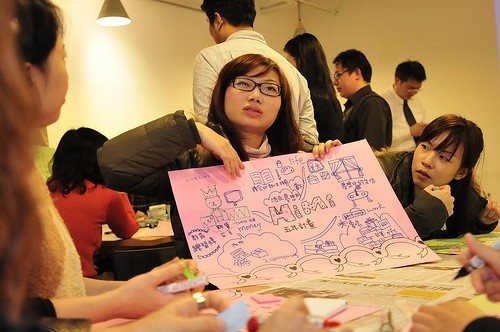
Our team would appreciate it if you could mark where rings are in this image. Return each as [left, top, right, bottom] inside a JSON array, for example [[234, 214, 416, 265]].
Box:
[[193, 292, 207, 311]]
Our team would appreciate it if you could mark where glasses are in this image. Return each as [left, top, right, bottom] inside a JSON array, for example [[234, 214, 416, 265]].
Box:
[[333, 66, 355, 79], [228, 77, 283, 97]]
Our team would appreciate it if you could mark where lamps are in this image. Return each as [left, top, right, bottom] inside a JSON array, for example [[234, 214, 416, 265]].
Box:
[[95, 0, 132, 27], [293, 0, 306, 36]]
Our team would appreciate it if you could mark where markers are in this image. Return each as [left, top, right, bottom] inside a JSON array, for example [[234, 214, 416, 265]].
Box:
[[450, 238, 500, 283], [430, 186, 446, 230], [248, 315, 341, 332]]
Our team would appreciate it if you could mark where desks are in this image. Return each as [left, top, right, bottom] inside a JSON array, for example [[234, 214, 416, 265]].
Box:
[[91, 228, 500, 332], [92, 220, 178, 283]]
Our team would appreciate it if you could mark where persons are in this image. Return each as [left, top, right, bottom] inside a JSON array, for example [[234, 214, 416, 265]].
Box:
[[0, 0, 500, 332]]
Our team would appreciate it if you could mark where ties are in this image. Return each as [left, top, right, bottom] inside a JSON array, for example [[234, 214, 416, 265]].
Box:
[[403, 100, 421, 145]]
